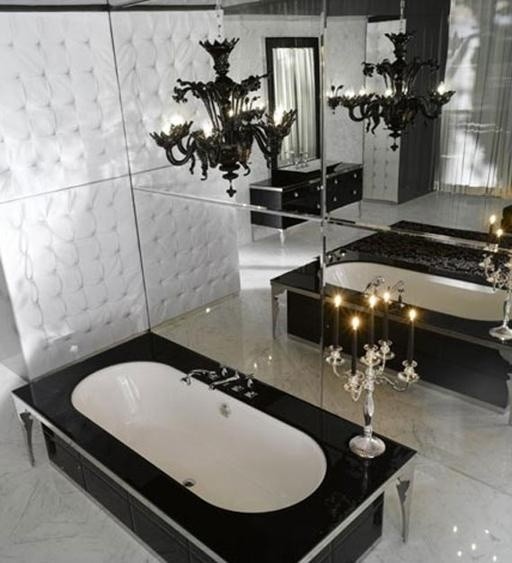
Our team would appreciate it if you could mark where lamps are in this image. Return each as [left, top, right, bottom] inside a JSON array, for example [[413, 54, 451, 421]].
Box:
[[479, 214, 512, 341], [149, 0, 297, 197], [324, 290, 421, 460], [327, 0, 456, 151]]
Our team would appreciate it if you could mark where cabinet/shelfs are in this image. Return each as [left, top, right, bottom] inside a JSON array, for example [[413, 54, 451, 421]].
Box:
[[249, 164, 363, 244]]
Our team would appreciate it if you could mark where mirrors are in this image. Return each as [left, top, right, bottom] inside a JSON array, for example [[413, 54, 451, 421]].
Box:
[[265, 36, 322, 171], [109, 0, 512, 495]]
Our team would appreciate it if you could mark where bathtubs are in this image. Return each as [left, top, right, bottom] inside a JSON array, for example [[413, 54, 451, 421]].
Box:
[[8, 330, 422, 562], [271, 248, 511, 417]]
